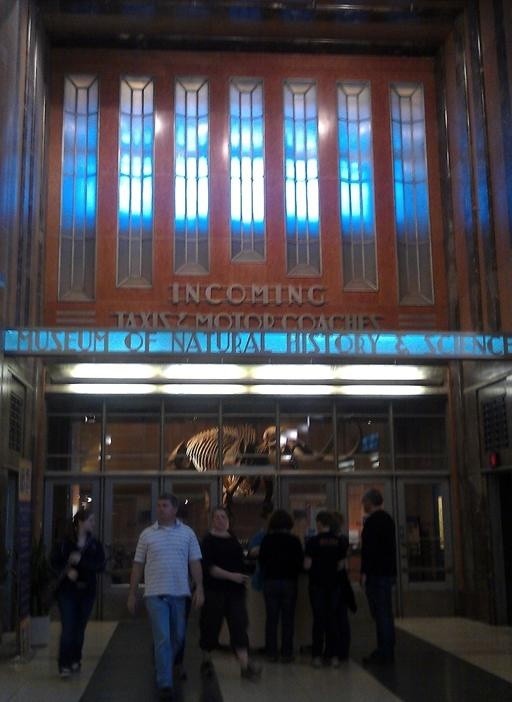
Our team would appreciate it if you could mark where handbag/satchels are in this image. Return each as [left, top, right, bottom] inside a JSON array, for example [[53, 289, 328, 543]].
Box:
[[251, 565, 271, 592]]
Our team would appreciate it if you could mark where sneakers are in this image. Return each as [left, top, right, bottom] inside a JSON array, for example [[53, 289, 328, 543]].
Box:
[[157, 645, 394, 702], [58, 663, 80, 679]]
